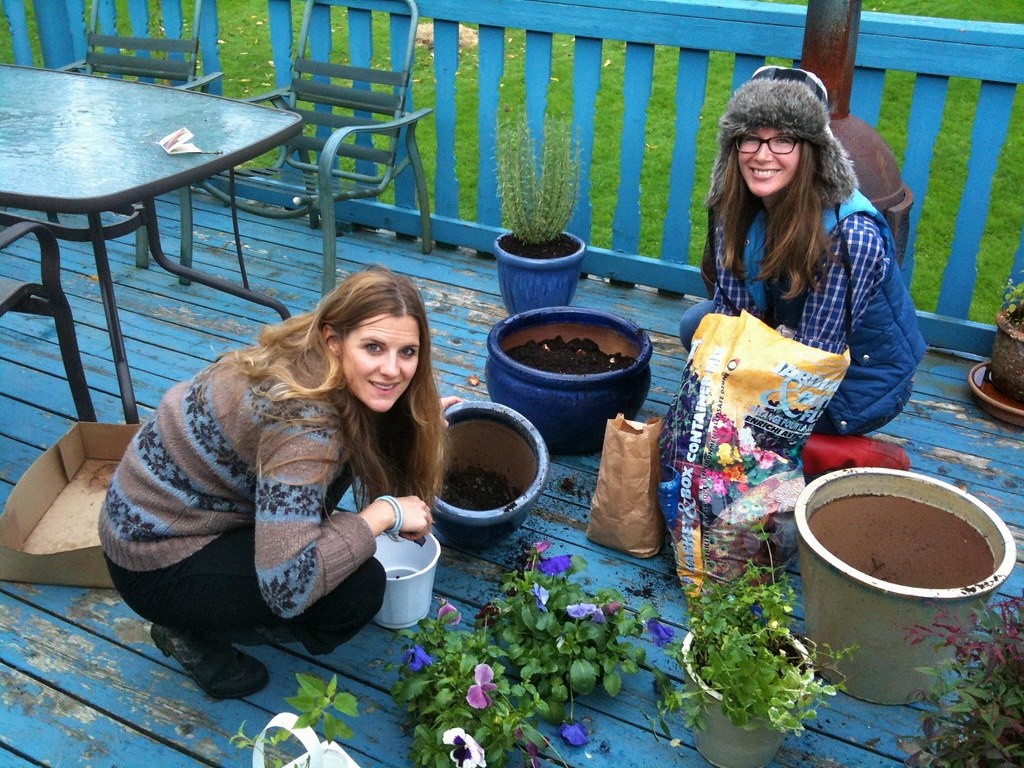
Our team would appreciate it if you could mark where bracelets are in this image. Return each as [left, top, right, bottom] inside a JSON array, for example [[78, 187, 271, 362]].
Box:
[[376, 495, 404, 536]]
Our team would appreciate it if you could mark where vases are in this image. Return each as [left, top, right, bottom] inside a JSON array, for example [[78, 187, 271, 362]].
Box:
[[795, 467, 1017, 706], [486, 306, 653, 453], [681, 625, 815, 768], [429, 400, 549, 550]]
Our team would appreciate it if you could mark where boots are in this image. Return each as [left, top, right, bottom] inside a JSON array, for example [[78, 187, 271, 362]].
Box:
[[802, 434, 910, 477]]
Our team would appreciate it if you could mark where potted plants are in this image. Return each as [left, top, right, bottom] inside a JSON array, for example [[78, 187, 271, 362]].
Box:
[[968, 271, 1024, 432], [490, 114, 585, 315]]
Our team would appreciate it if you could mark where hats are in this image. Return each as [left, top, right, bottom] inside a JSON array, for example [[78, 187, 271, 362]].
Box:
[[703, 65, 860, 208]]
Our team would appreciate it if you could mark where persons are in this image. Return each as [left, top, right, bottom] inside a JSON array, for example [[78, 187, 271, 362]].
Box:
[[99, 264, 468, 701], [681, 64, 927, 475]]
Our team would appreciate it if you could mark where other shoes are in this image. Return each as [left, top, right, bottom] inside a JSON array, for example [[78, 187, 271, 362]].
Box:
[[242, 615, 297, 646], [150, 623, 268, 699]]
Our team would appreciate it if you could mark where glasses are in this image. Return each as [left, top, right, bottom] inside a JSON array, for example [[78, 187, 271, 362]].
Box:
[[737, 130, 804, 154]]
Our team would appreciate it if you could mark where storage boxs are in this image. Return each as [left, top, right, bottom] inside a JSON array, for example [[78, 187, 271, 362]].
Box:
[[0, 421, 141, 589]]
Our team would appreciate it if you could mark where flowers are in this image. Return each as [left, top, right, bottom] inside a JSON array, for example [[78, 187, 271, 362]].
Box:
[[642, 523, 858, 743], [383, 539, 646, 768]]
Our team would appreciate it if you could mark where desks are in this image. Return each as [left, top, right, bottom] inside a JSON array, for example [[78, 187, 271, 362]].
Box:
[[0, 64, 303, 425]]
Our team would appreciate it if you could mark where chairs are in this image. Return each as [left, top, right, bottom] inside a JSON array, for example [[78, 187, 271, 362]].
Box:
[[0, 221, 97, 422], [54, 0, 202, 270], [178, 0, 434, 296]]
